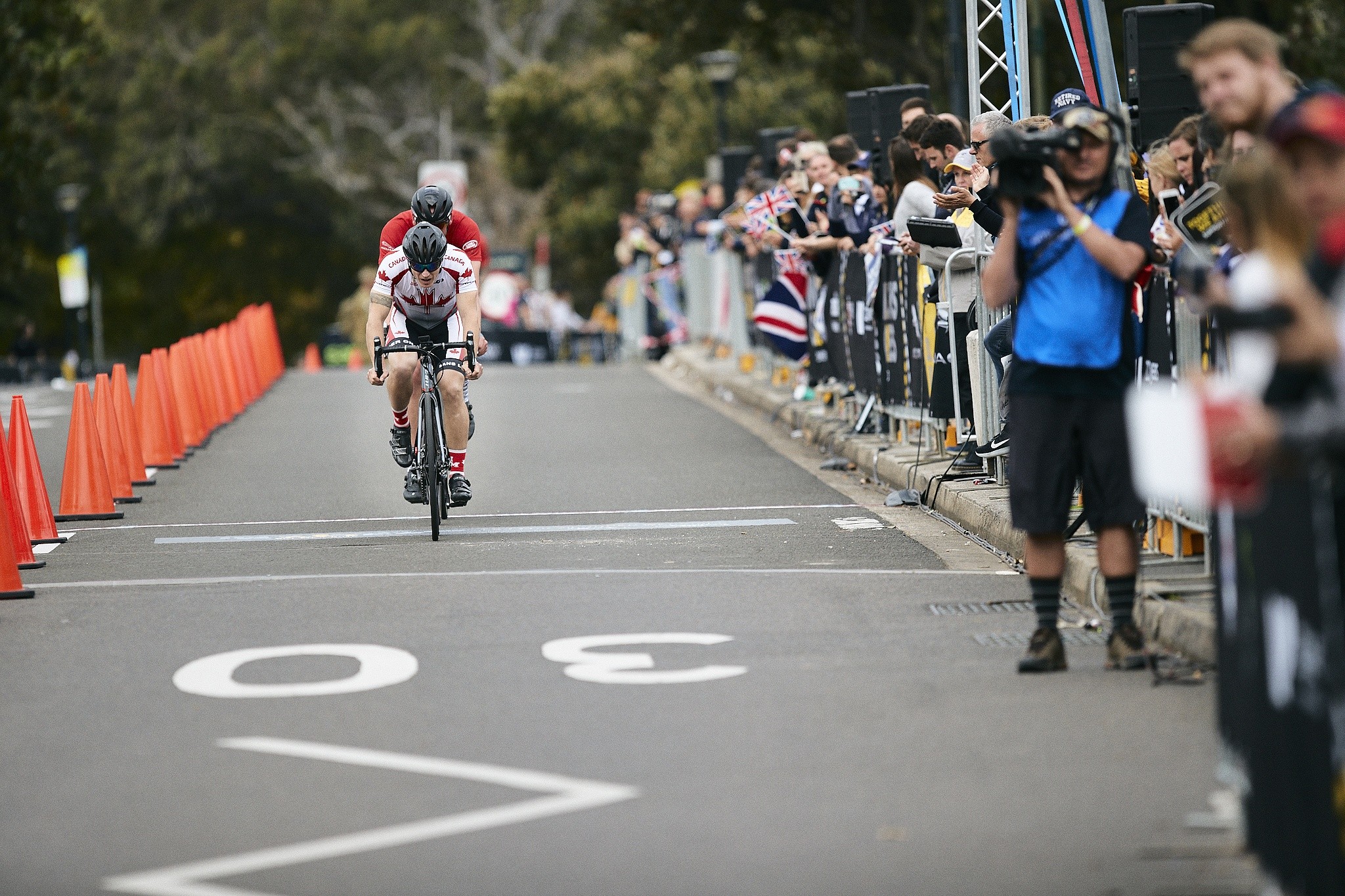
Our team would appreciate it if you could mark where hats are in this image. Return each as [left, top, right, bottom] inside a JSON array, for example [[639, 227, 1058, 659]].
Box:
[[1049, 88, 1091, 119], [944, 148, 978, 173], [1062, 106, 1121, 144], [1273, 91, 1345, 148], [846, 152, 872, 170]]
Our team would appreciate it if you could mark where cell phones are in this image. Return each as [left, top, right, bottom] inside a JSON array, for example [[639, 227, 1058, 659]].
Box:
[[1159, 189, 1181, 223]]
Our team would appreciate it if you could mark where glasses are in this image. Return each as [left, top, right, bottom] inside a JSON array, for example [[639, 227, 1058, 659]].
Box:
[[971, 139, 989, 150], [409, 260, 442, 273], [418, 222, 446, 231]]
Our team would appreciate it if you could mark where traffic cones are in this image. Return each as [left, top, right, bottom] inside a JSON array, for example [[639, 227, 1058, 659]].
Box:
[[52, 378, 125, 523], [305, 342, 325, 374], [347, 346, 366, 373], [110, 362, 157, 487], [152, 301, 286, 462], [91, 373, 143, 504], [6, 394, 67, 544], [133, 353, 180, 470], [0, 458, 35, 600], [0, 415, 48, 571]]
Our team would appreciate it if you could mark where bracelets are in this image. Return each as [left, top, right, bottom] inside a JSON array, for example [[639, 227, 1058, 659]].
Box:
[[1070, 212, 1091, 239]]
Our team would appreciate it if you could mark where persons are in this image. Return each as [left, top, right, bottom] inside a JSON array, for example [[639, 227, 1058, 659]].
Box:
[[978, 102, 1159, 675], [617, 15, 1344, 896], [365, 220, 483, 503], [379, 184, 488, 506]]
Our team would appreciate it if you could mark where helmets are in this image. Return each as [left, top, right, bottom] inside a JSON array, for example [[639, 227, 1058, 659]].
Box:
[[411, 185, 453, 223], [402, 222, 448, 262]]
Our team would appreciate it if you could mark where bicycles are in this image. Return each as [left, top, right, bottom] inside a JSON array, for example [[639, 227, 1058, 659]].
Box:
[[369, 320, 479, 541]]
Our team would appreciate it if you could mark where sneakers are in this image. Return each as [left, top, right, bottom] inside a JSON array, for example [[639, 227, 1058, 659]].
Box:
[[449, 473, 472, 501], [1103, 626, 1151, 670], [464, 400, 475, 441], [403, 469, 422, 503], [1018, 630, 1068, 671], [974, 422, 1011, 458], [389, 421, 414, 468]]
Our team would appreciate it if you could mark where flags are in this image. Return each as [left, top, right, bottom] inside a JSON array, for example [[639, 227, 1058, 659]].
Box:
[[751, 266, 811, 363], [772, 248, 808, 273], [867, 219, 895, 237], [741, 184, 804, 275]]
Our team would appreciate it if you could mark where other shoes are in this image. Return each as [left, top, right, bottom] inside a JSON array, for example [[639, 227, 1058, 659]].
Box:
[[946, 441, 973, 456], [952, 459, 983, 472]]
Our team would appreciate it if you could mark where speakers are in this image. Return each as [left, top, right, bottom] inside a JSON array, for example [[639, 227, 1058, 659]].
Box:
[[845, 91, 870, 150], [759, 127, 795, 181], [867, 84, 930, 184]]
